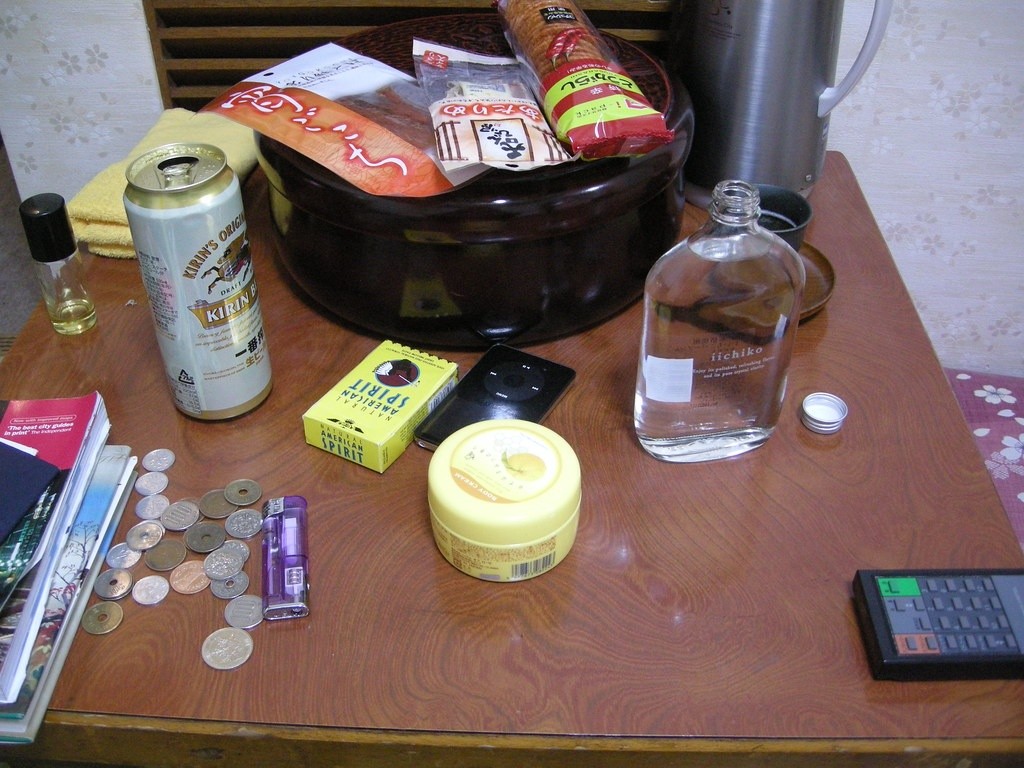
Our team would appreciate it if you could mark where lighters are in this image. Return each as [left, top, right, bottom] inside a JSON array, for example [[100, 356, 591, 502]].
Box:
[[262, 493, 311, 622]]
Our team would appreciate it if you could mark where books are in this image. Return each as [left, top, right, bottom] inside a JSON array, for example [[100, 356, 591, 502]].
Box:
[[0, 391, 113, 702], [0, 425, 137, 746]]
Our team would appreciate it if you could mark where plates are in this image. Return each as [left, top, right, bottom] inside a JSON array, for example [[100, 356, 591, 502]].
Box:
[[796, 241, 836, 321]]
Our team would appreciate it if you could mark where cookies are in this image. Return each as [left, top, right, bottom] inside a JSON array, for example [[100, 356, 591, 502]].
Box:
[[504, 0, 607, 81]]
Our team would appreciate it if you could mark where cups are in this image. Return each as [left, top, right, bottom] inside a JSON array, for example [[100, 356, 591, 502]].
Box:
[[749, 184, 813, 253]]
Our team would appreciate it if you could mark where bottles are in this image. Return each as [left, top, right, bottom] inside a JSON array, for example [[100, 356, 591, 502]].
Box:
[[632, 179, 807, 463]]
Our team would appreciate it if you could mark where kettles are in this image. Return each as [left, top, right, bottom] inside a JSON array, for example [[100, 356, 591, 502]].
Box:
[[667, 0, 894, 210]]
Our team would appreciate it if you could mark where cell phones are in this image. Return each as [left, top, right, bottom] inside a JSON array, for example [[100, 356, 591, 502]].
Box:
[[412, 344, 578, 452]]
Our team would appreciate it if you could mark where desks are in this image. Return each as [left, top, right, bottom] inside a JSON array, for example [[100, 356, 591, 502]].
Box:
[[0, 149, 1024, 768]]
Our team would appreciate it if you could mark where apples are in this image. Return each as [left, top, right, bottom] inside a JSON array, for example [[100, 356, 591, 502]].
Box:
[[502, 452, 547, 481]]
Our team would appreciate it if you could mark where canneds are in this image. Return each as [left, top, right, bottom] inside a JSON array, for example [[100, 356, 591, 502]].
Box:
[[122, 142, 273, 422]]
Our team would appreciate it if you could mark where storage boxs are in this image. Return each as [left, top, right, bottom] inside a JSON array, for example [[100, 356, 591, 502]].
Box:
[[301, 340, 460, 474]]
